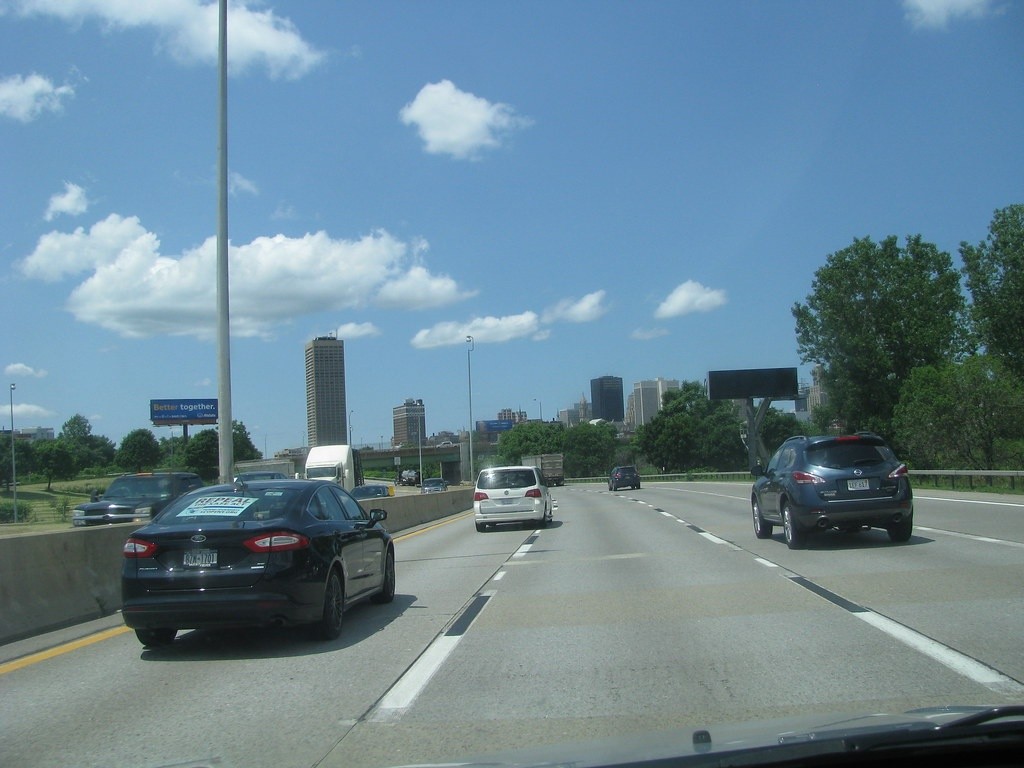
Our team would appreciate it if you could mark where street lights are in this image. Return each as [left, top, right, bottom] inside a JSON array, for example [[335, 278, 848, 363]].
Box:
[[466, 335, 474, 485], [417, 413, 422, 490], [265, 432, 267, 459], [533, 398, 542, 423], [349, 410, 354, 448], [9, 383, 19, 523], [216, 0, 234, 486]]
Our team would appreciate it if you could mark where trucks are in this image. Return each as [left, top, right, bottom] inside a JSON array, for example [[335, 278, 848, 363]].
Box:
[[295, 444, 364, 493], [394, 469, 424, 486], [521, 453, 564, 486]]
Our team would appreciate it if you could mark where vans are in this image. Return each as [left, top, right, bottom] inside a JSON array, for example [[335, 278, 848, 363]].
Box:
[[473, 466, 554, 532]]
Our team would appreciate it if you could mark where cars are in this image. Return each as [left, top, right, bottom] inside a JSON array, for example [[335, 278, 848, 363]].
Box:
[[608, 465, 641, 491], [233, 471, 288, 484], [435, 441, 453, 449], [750, 430, 915, 550], [69, 473, 207, 528], [350, 485, 395, 501], [119, 478, 396, 646], [416, 478, 449, 494]]
